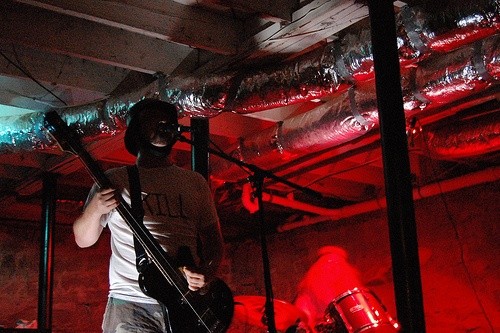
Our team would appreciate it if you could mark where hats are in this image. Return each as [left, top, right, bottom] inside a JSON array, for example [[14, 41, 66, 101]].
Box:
[[123, 98, 178, 158]]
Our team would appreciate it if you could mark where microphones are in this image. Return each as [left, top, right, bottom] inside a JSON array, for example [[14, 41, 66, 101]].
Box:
[[159, 120, 193, 133]]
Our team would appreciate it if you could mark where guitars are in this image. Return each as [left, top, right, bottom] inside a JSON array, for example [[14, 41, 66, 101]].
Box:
[[43, 108, 235, 333]]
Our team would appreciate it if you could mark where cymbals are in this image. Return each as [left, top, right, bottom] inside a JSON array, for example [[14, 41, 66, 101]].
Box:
[[233, 294, 309, 332]]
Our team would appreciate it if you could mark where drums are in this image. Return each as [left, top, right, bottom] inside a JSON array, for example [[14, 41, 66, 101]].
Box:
[[323, 286, 398, 333]]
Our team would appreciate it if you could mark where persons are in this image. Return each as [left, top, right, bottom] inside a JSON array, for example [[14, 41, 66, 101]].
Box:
[[73, 96, 224, 333]]
